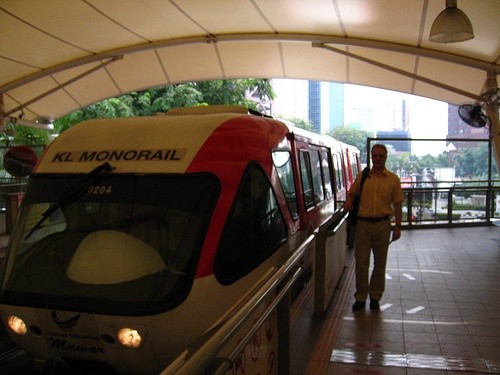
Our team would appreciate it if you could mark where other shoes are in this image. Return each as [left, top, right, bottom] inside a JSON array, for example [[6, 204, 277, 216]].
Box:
[[370, 300, 380, 310], [352, 301, 365, 311]]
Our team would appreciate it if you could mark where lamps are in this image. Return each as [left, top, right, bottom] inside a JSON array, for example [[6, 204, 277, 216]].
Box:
[[429, 0, 475, 43]]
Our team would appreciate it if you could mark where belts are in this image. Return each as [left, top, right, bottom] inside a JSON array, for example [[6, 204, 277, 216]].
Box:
[[358, 216, 389, 223]]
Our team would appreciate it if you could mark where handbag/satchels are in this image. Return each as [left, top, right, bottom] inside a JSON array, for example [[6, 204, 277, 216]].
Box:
[[345, 166, 370, 246]]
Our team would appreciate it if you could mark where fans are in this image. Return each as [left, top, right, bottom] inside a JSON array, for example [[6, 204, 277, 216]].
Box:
[[457, 103, 493, 140]]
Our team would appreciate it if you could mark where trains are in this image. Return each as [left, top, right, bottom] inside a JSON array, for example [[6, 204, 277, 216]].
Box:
[[0, 103, 362, 375]]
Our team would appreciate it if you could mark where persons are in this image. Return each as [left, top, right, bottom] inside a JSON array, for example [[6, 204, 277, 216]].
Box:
[[345, 143, 404, 314]]
